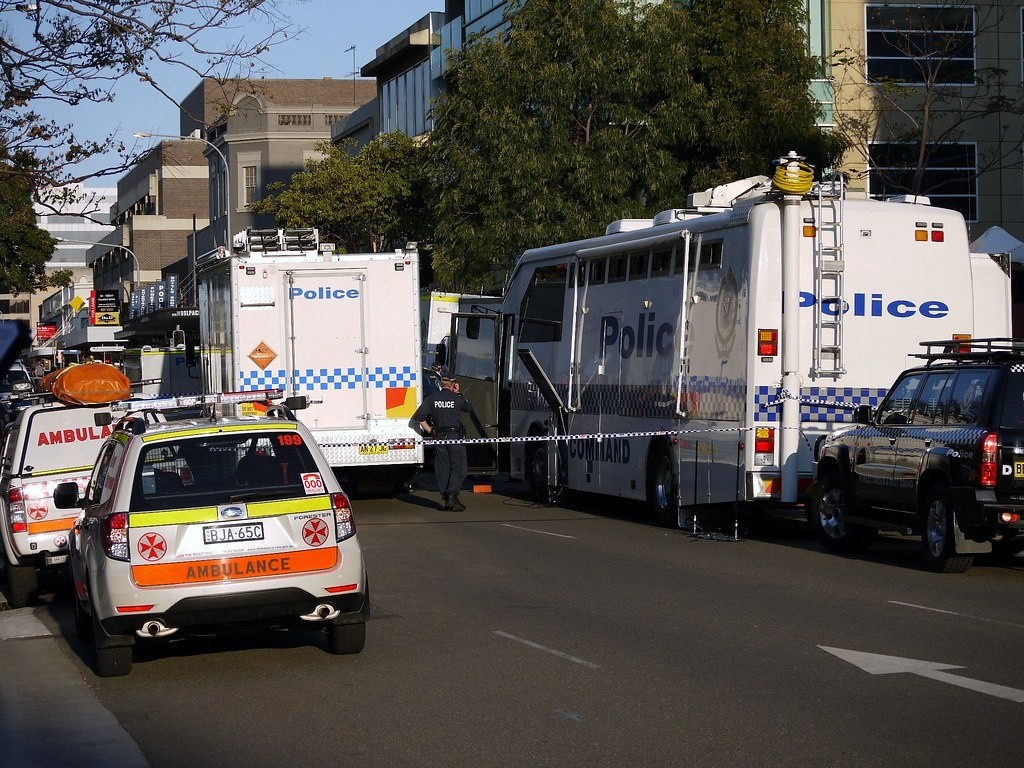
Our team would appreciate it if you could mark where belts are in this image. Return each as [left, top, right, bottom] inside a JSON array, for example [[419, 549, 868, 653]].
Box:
[[432, 426, 461, 433]]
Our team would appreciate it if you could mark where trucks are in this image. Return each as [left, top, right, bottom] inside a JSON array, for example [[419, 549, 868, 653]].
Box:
[[193, 228, 426, 490], [412, 287, 510, 483], [448, 160, 970, 526]]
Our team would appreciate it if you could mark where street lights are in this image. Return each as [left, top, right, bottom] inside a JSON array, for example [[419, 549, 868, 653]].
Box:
[[53, 237, 140, 288], [132, 130, 232, 252]]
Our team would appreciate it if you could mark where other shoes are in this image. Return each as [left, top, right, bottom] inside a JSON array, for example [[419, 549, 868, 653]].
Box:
[[445, 492, 466, 511]]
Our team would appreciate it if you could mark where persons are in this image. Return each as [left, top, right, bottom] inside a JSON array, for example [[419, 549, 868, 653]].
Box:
[[412, 373, 474, 516], [30, 353, 121, 382], [431, 363, 442, 374]]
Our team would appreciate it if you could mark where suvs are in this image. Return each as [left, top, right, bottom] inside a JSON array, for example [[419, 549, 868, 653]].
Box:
[[54, 393, 370, 677], [809, 337, 1024, 573], [0, 336, 208, 612]]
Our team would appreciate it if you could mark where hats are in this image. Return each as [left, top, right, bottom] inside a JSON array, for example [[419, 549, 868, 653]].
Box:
[[441, 372, 457, 382], [431, 362, 442, 371]]
